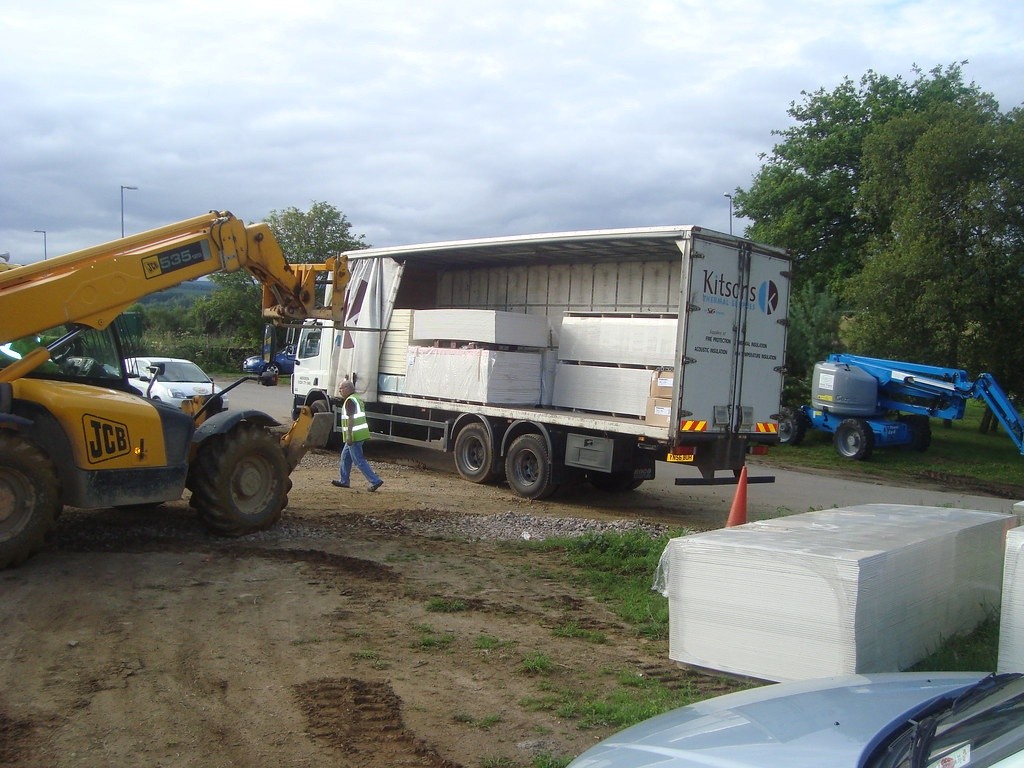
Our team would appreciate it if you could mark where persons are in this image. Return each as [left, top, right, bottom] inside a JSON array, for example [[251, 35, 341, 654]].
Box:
[[331, 380, 383, 492]]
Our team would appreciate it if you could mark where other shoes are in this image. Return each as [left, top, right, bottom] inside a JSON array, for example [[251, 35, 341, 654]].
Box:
[[367, 479, 384, 492], [331, 480, 351, 488]]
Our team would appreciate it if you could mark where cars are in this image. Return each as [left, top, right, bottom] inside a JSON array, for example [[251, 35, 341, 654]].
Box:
[[242, 343, 297, 377], [564, 668, 1024, 767], [126, 357, 229, 415]]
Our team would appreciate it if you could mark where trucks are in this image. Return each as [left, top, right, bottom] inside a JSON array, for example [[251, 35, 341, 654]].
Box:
[[285, 224, 796, 501]]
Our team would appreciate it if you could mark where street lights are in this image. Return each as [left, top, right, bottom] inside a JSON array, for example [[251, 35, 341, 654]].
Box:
[[34, 230, 46, 260], [120, 185, 138, 238]]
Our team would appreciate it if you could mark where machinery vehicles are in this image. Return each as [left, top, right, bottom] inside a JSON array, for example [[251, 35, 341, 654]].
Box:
[[779, 353, 1024, 459], [0, 209, 351, 572]]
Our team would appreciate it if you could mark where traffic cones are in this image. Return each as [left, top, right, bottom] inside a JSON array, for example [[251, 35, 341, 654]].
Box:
[[724, 463, 747, 525]]
